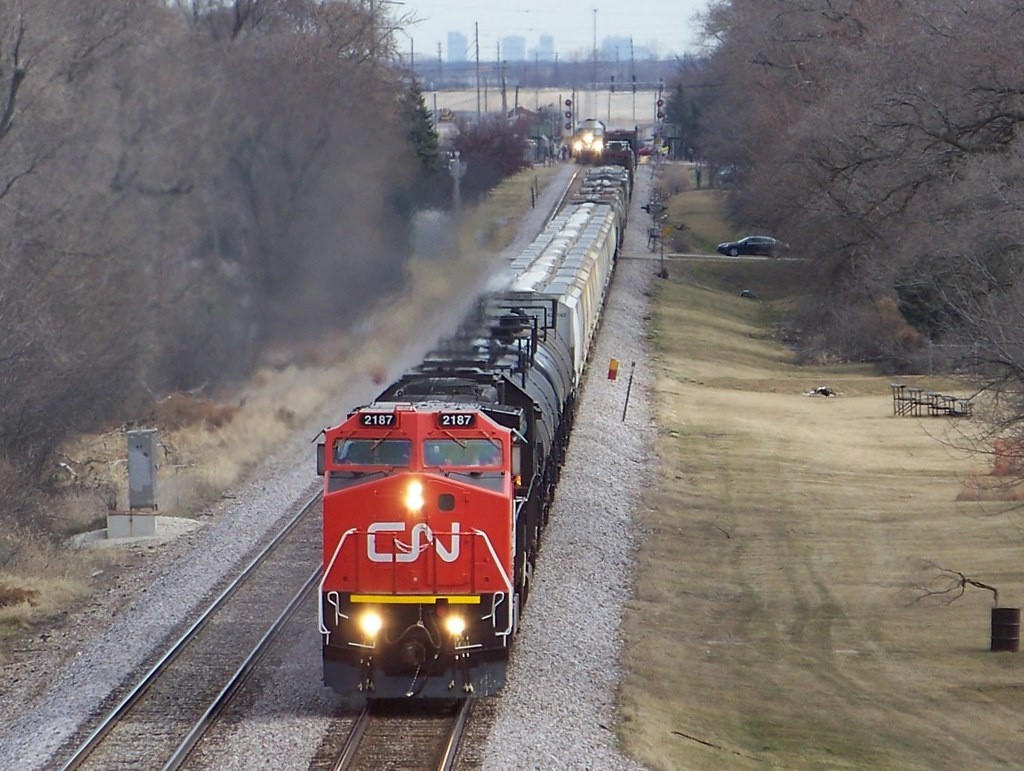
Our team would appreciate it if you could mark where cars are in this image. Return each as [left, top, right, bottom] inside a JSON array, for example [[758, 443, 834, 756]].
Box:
[[715, 236, 791, 260]]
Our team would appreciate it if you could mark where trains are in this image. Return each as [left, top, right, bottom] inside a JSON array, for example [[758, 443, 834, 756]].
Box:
[[307, 136, 640, 713], [572, 117, 606, 163]]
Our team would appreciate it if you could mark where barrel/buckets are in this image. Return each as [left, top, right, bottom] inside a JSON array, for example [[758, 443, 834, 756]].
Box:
[[989, 607, 1021, 652]]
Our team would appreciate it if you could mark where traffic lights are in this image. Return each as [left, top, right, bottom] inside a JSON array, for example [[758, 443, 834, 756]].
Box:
[[565, 123, 570, 128], [566, 112, 571, 117], [565, 100, 571, 106], [658, 100, 663, 106], [657, 112, 663, 118]]
